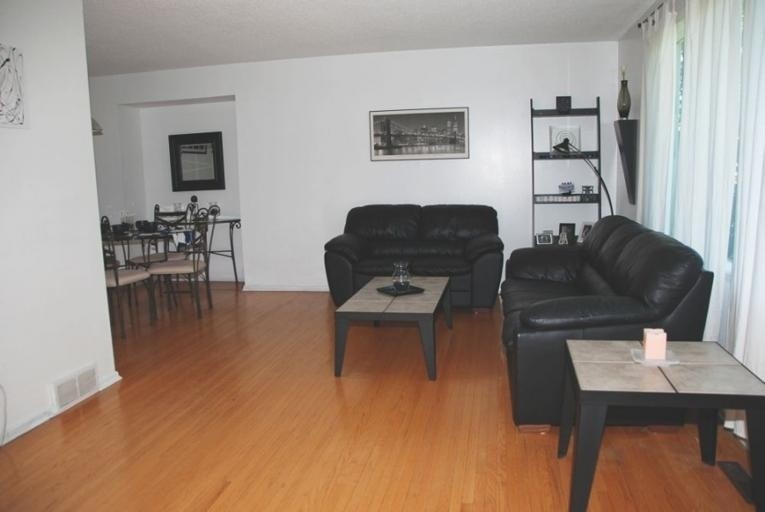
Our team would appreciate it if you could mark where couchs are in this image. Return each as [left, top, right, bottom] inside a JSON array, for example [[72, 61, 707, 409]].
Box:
[[499, 214, 714, 427], [325, 204, 504, 312]]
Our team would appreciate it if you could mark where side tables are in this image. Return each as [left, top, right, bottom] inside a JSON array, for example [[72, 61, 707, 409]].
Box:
[[558, 338, 765, 512]]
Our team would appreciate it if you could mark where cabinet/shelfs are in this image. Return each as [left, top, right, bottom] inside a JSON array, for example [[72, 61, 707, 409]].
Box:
[[531, 97, 601, 243]]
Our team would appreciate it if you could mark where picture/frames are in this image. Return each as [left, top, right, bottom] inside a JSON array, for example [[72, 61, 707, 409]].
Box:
[[369, 107, 470, 160]]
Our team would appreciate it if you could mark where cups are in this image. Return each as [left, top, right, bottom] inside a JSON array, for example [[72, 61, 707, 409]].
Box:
[[556, 96, 571, 115], [174, 202, 182, 213]]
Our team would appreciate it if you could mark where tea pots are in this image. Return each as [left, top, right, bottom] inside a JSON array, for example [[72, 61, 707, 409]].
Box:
[[391, 261, 411, 291]]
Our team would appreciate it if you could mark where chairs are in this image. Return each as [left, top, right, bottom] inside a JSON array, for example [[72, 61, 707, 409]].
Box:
[[100, 194, 240, 340]]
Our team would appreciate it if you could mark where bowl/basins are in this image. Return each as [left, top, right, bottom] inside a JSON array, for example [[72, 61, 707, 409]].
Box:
[[112, 219, 157, 235]]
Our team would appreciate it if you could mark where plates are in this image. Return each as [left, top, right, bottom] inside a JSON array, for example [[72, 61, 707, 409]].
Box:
[[378, 282, 422, 294], [631, 346, 681, 366]]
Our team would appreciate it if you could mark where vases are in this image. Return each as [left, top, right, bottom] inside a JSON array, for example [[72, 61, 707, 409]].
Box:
[[616, 65, 631, 116]]
